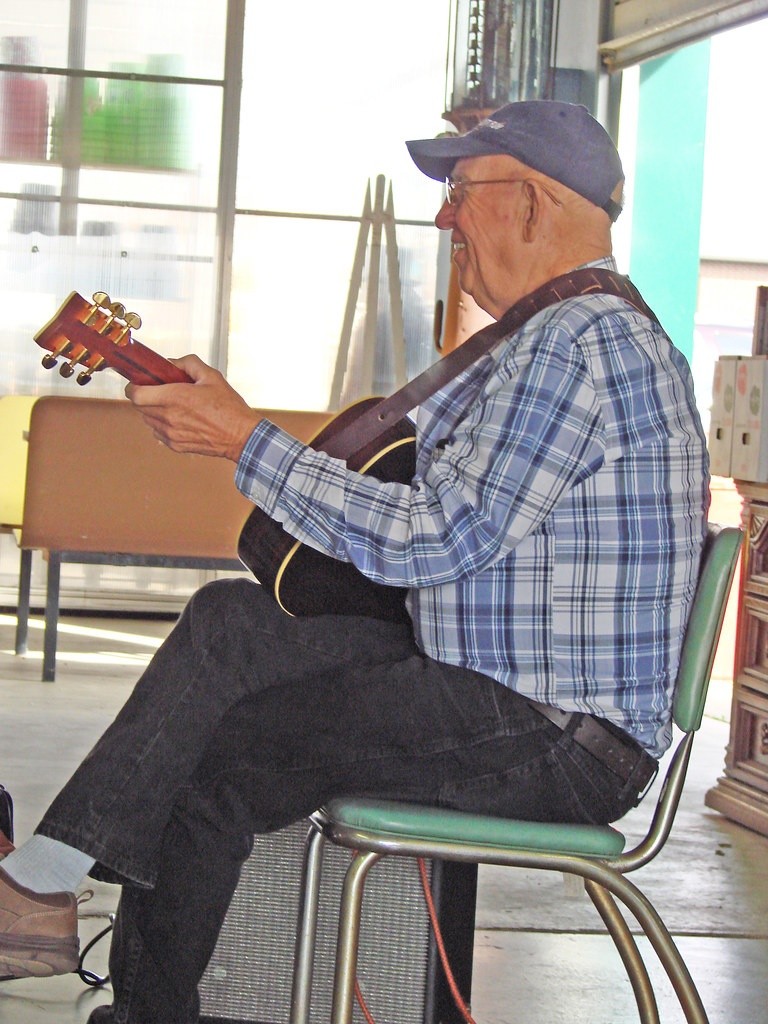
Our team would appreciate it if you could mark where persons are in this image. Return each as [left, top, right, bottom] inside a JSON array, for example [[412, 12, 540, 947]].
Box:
[[0, 98, 710, 1024]]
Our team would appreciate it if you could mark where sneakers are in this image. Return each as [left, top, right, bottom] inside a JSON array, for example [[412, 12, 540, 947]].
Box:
[[0, 867, 95, 978]]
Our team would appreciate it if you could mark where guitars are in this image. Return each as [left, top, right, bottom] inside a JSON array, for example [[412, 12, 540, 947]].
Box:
[[30, 286, 419, 620]]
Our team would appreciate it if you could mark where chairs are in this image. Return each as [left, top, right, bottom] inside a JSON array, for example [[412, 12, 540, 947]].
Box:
[[291, 529, 746, 1024]]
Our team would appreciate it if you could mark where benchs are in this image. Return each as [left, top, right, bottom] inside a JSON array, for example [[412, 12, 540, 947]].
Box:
[[11, 394, 332, 686]]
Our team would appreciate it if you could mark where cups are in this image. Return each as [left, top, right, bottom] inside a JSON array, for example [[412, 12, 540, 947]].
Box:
[[0, 35, 194, 170]]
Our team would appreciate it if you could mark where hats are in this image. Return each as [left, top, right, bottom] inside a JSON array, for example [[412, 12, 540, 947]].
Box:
[[405, 99, 623, 221]]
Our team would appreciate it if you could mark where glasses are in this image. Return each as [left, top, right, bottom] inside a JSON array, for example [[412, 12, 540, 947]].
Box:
[[445, 176, 564, 208]]
